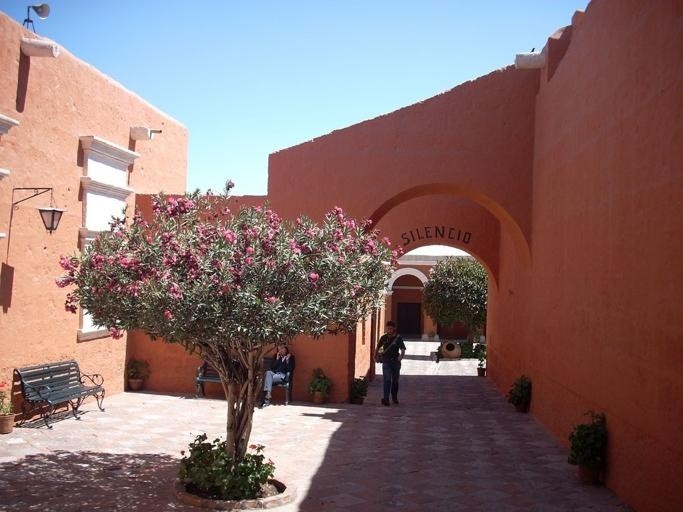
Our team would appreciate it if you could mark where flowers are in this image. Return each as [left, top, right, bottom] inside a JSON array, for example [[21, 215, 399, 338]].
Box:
[[0, 383, 15, 415]]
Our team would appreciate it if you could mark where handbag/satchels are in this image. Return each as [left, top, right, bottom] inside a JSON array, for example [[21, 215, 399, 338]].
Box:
[[374, 353, 384, 363]]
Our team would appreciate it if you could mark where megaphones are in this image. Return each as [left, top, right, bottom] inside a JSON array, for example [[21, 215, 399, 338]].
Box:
[[31, 3, 50, 20]]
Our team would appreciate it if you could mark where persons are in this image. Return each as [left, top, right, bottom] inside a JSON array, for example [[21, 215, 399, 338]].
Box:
[[262, 342, 295, 407], [374, 320, 406, 406]]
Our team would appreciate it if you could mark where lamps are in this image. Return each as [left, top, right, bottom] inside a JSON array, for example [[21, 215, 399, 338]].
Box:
[[12, 187, 66, 234], [22, 3, 50, 33]]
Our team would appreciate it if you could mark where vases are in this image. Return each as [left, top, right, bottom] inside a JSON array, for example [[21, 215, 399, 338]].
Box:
[[0, 414, 15, 433]]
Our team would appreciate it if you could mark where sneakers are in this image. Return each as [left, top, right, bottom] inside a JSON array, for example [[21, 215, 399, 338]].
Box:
[[259, 392, 272, 408], [381, 397, 400, 406]]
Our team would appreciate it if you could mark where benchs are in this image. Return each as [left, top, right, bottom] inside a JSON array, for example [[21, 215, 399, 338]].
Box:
[[193, 355, 295, 406], [14, 359, 107, 429]]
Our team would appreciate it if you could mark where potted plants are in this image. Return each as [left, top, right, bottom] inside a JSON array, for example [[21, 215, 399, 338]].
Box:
[[567, 409, 607, 483], [307, 368, 331, 404], [476, 349, 487, 376], [126, 359, 150, 390], [504, 375, 532, 413], [350, 375, 369, 405]]
